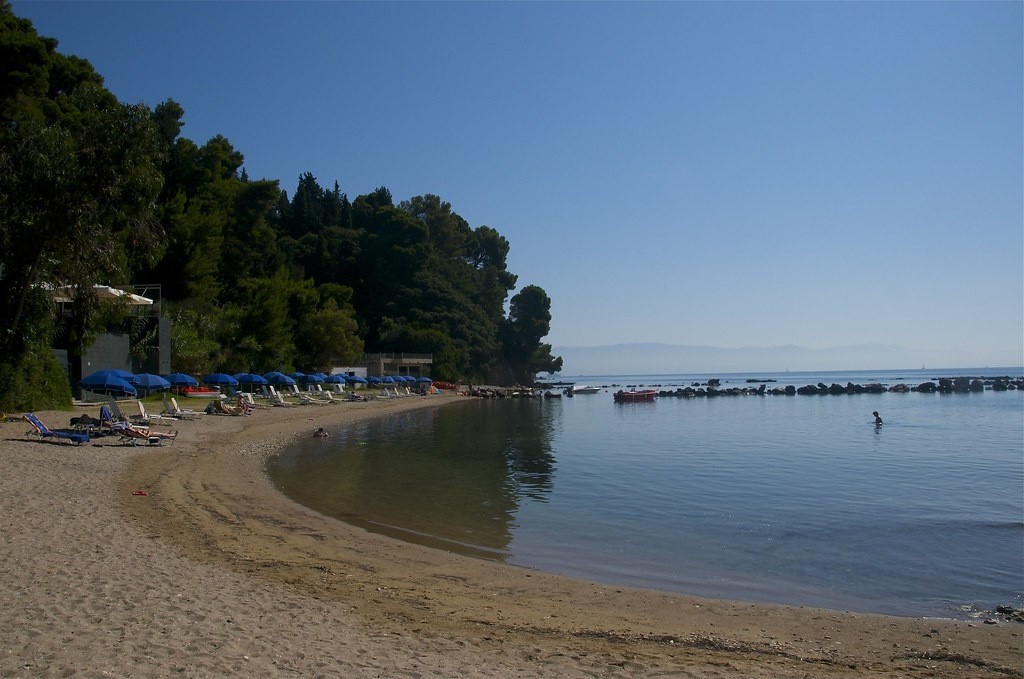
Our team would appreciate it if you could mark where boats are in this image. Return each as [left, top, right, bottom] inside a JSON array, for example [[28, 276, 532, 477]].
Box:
[[613, 389, 656, 400], [546, 394, 561, 398], [566, 387, 601, 394], [548, 381, 576, 385]]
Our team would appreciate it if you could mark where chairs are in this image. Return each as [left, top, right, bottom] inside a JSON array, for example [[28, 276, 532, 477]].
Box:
[[162, 398, 196, 421], [276, 391, 293, 408], [261, 386, 270, 398], [327, 390, 349, 403], [98, 406, 150, 434], [304, 394, 331, 405], [317, 384, 335, 396], [246, 393, 274, 410], [171, 397, 207, 419], [109, 421, 179, 447], [137, 400, 179, 426], [311, 385, 322, 396], [22, 412, 90, 447], [335, 384, 421, 402], [270, 386, 285, 398], [213, 400, 244, 416], [227, 386, 245, 401], [293, 385, 308, 398], [109, 401, 151, 427]]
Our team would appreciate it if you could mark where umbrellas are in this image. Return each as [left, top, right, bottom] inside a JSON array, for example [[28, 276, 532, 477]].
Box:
[[203, 373, 239, 398], [135, 373, 171, 411], [78, 374, 136, 432], [92, 368, 136, 384], [232, 370, 432, 397], [118, 412, 146, 423]]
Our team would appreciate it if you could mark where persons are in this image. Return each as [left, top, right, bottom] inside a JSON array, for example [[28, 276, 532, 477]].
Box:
[[218, 400, 244, 416], [873, 411, 883, 423], [466, 384, 504, 399], [237, 393, 252, 416], [123, 421, 178, 438], [163, 372, 198, 412], [314, 428, 323, 437], [78, 413, 114, 427]]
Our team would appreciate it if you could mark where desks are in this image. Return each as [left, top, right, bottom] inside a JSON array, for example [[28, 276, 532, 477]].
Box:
[[74, 423, 95, 437]]
